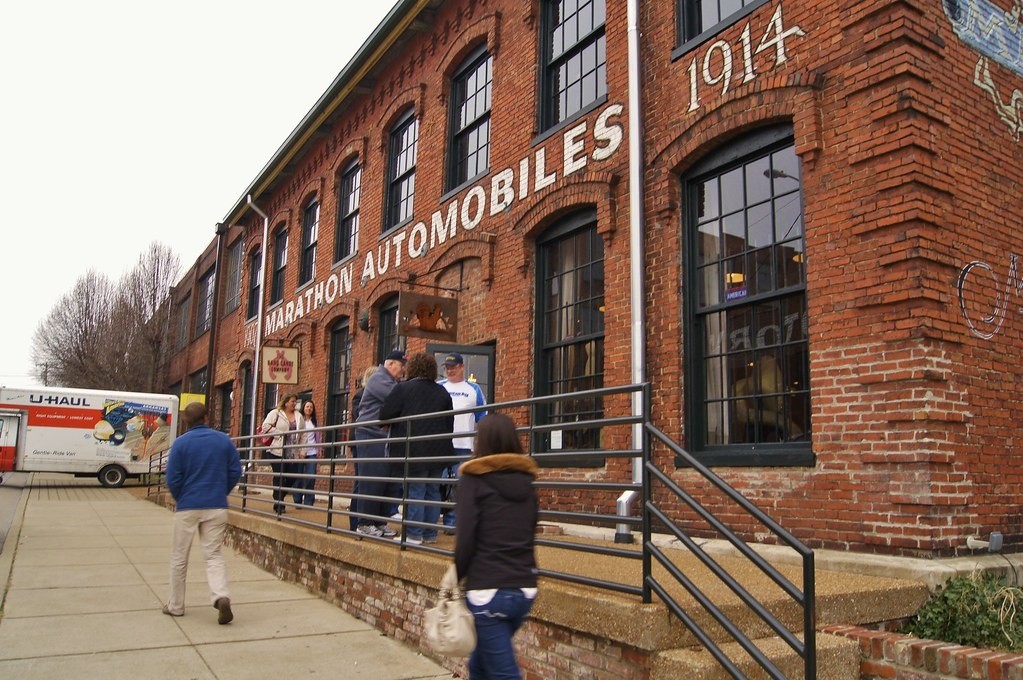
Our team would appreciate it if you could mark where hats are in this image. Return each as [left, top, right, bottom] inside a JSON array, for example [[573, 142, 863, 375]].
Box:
[[386, 350, 408, 363], [441, 354, 463, 367]]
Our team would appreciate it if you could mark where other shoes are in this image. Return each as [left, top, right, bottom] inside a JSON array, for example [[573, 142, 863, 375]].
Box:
[[275, 508, 286, 512], [443, 525, 455, 535], [391, 513, 403, 520], [217, 597, 233, 624], [163, 603, 184, 616], [422, 528, 438, 543], [393, 528, 424, 545]]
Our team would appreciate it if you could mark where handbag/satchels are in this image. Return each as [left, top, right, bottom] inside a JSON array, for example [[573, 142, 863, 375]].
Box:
[[423, 564, 478, 657], [257, 409, 280, 445]]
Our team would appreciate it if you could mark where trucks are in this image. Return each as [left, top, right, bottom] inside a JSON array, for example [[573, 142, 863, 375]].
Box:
[[0, 387, 179, 488]]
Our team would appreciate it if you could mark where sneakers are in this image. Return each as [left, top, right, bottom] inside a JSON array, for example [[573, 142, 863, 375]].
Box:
[[375, 523, 397, 536], [356, 524, 383, 538]]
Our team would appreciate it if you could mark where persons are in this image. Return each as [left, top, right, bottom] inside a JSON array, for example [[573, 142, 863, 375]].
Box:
[[348, 349, 486, 544], [163, 402, 242, 624], [262, 393, 307, 514], [292, 401, 322, 509], [454, 414, 539, 680]]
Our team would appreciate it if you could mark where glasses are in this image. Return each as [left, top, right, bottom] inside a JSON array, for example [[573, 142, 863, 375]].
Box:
[[443, 365, 461, 370]]
